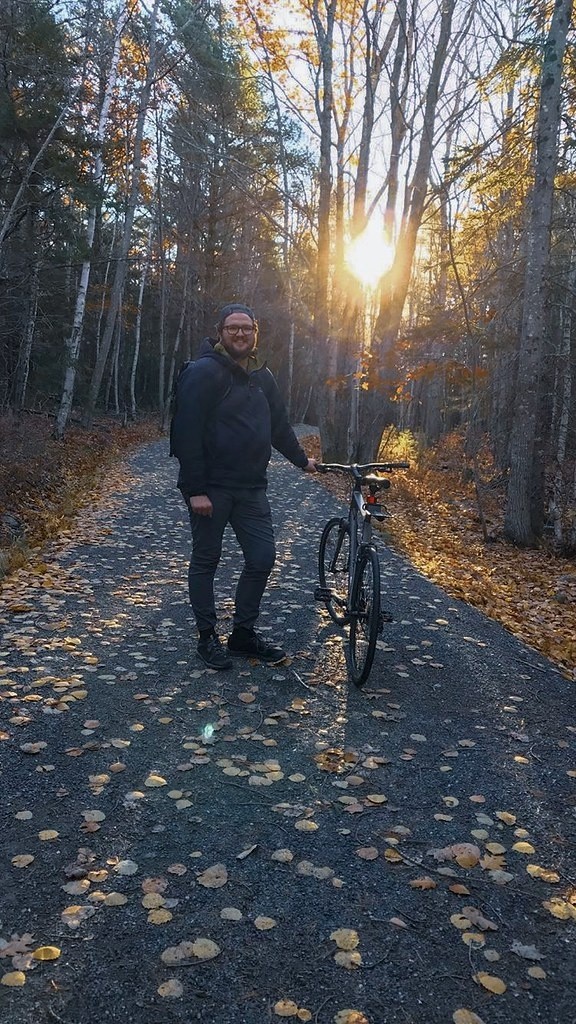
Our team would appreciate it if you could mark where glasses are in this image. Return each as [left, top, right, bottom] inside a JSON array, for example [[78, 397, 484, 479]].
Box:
[[221, 325, 256, 336]]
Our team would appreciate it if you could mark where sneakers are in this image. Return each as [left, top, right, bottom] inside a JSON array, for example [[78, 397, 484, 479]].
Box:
[[195, 629, 231, 670], [227, 634, 286, 661]]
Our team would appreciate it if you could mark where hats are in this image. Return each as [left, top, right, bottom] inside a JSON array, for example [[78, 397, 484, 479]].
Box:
[[219, 303, 255, 324]]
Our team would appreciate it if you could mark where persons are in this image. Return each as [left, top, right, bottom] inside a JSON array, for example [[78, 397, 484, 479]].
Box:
[[170, 304, 319, 670]]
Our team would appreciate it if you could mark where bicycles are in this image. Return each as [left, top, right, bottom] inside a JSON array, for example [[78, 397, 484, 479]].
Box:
[[301, 459, 410, 688]]
